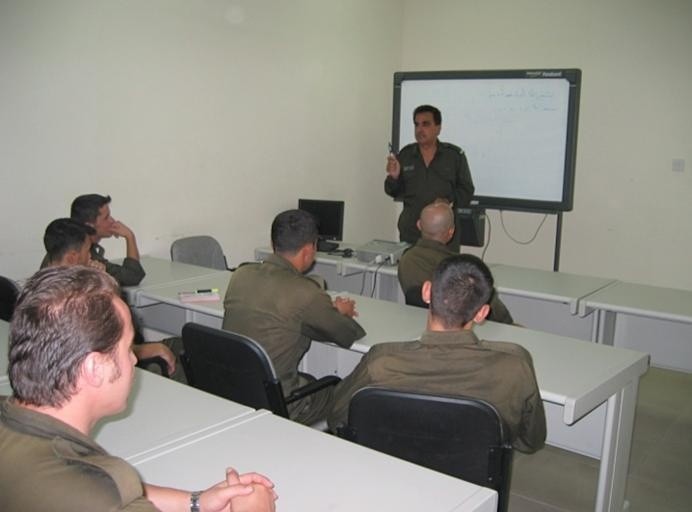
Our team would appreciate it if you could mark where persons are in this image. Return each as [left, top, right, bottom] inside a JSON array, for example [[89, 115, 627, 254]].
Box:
[[44, 218, 189, 385], [221, 209, 366, 427], [39, 194, 146, 286], [384, 105, 474, 254], [324, 254, 546, 511], [398, 199, 514, 325], [0, 265, 279, 512]]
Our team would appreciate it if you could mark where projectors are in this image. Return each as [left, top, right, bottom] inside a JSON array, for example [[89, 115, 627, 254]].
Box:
[[356, 239, 412, 266]]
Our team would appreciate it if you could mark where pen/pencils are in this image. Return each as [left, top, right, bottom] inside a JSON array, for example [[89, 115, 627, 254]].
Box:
[[177, 288, 219, 295]]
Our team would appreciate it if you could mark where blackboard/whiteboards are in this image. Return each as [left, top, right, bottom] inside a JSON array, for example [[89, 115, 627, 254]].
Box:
[[388, 66, 582, 214]]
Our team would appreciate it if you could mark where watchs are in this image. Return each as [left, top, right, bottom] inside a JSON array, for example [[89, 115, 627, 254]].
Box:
[[191, 490, 204, 512]]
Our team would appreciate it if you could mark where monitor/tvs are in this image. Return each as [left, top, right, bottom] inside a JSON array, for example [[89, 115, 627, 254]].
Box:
[[299, 200, 344, 252]]
